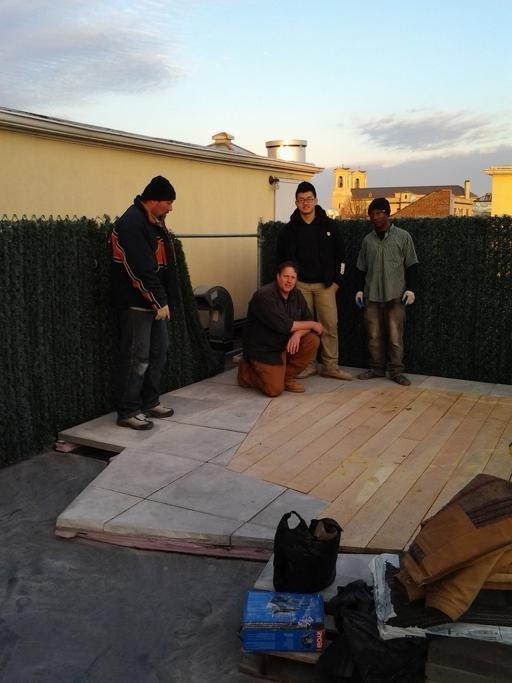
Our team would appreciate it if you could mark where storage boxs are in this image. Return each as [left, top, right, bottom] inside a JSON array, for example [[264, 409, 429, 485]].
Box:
[[243, 590, 324, 654]]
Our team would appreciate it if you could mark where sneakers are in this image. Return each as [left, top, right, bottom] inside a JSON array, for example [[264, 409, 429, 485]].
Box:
[[285, 381, 305, 393], [238, 358, 251, 388], [320, 366, 352, 381], [143, 403, 174, 417], [388, 372, 410, 386], [118, 414, 153, 430], [359, 369, 385, 380], [295, 363, 318, 379]]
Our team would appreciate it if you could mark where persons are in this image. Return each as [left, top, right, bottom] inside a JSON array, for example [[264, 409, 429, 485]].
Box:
[[107, 175, 177, 431], [236, 257, 324, 398], [351, 196, 420, 386], [274, 180, 353, 381]]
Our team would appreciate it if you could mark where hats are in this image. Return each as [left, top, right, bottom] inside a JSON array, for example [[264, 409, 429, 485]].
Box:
[[143, 176, 175, 201], [368, 198, 390, 215]]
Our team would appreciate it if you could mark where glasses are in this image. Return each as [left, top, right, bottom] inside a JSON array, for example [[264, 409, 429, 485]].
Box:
[[297, 198, 314, 203]]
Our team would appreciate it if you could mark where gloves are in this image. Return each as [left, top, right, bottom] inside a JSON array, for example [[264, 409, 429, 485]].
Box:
[[355, 291, 365, 309], [402, 290, 416, 305]]
